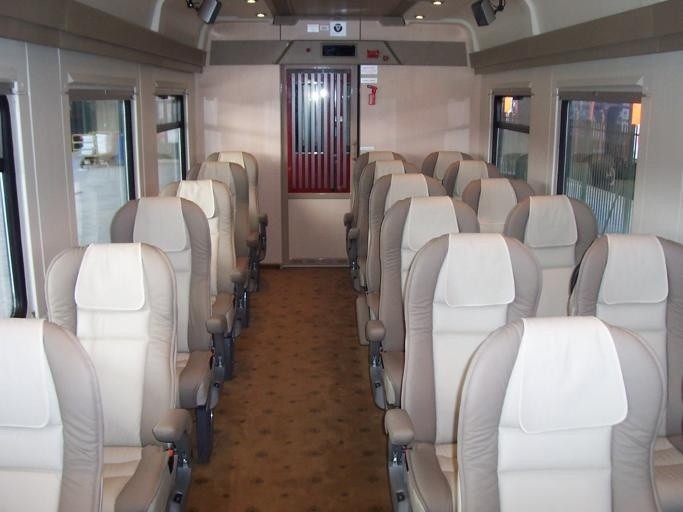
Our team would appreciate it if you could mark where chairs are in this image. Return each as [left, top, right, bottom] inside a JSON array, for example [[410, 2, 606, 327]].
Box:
[[502, 194, 601, 319], [185, 160, 263, 309], [340, 151, 537, 347], [44, 240, 196, 512], [452, 311, 670, 512], [563, 231, 683, 512], [1, 314, 108, 512], [383, 231, 546, 510], [111, 194, 226, 466], [160, 178, 252, 380], [365, 195, 482, 410], [207, 150, 269, 290]]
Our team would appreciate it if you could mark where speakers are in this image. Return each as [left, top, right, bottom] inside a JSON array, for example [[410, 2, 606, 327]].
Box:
[[197, 0, 221, 25], [471, 0, 496, 27]]
